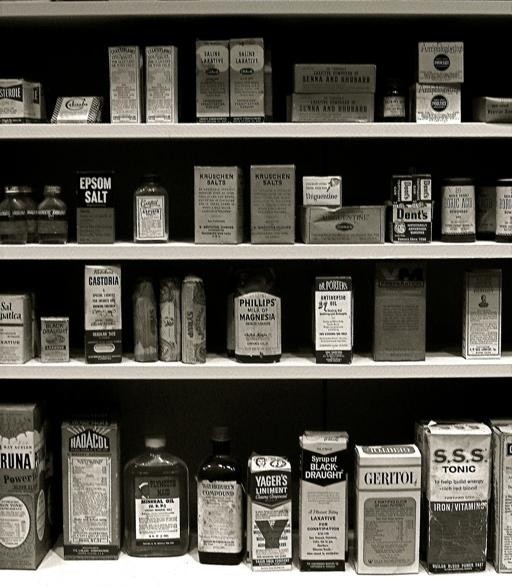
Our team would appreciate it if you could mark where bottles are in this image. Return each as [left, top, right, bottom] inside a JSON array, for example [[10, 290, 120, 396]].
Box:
[[196, 424, 247, 565], [232, 274, 283, 364], [119, 430, 191, 558], [383, 88, 408, 122], [0, 186, 69, 244], [132, 173, 170, 244]]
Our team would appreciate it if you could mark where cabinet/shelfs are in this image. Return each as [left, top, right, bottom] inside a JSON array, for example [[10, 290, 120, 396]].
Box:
[[1, 0, 512, 587]]
